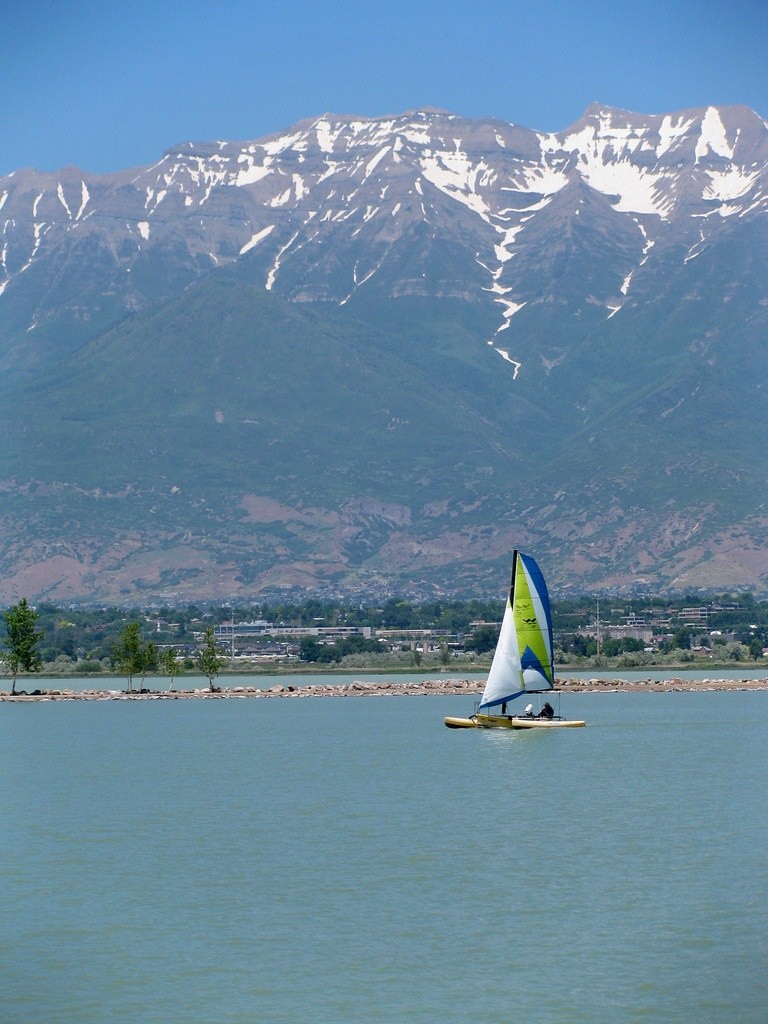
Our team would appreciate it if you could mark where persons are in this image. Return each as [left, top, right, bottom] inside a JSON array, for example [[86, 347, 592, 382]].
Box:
[[538, 702, 554, 719]]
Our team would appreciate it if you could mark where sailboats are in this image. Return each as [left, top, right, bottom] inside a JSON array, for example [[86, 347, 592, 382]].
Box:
[[442, 549, 588, 730]]
